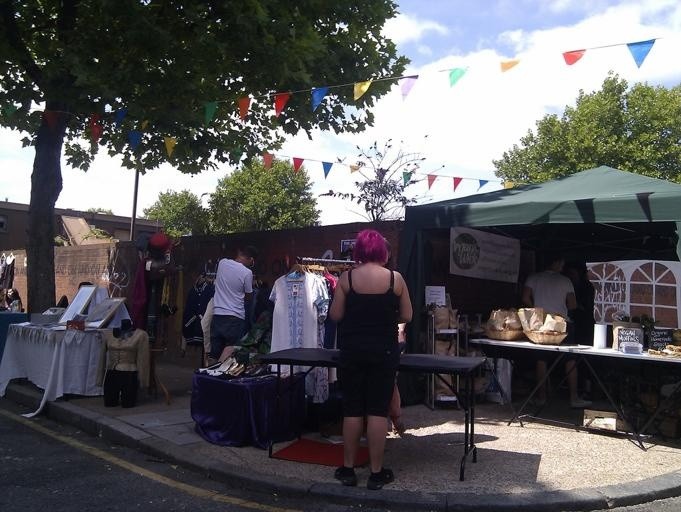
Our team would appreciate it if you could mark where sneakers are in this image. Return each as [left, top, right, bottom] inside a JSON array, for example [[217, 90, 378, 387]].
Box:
[[367, 467, 394, 490], [570, 399, 592, 407], [523, 397, 549, 407], [336, 466, 357, 486]]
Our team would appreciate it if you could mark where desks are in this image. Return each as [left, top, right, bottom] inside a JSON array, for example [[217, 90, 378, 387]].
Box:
[[469, 338, 681, 452], [254, 346, 491, 482], [0, 319, 138, 419]]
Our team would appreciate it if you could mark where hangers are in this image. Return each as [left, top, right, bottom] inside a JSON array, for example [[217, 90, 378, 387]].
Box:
[[200, 271, 267, 289], [0, 251, 17, 258], [288, 257, 358, 275]]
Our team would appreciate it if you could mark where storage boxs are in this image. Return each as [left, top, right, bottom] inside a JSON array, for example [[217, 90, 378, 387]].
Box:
[[190, 361, 307, 449]]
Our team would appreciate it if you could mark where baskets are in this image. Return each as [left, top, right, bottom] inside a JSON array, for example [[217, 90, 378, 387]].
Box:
[[486, 329, 523, 340], [523, 330, 568, 345]]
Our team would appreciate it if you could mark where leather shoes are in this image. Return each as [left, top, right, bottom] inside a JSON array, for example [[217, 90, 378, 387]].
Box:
[[194, 356, 271, 379]]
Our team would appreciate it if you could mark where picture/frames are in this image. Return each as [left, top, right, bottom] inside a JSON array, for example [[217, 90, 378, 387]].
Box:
[[84, 295, 128, 329], [58, 284, 99, 325]]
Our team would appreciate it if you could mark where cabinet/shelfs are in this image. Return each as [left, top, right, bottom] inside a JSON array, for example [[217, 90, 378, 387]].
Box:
[[426, 313, 463, 410]]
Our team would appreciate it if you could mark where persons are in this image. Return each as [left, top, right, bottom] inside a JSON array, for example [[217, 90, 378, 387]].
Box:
[[1, 288, 22, 310], [209, 244, 258, 365], [96, 318, 150, 408], [328, 229, 413, 492], [521, 250, 594, 407], [381, 232, 418, 444]]
